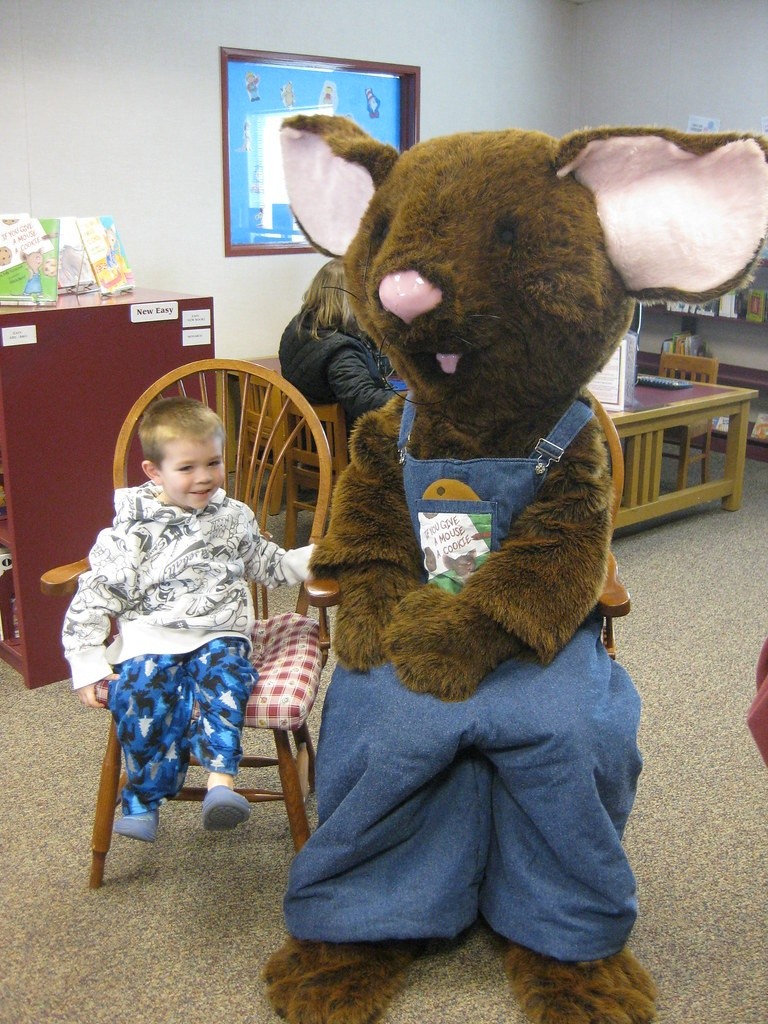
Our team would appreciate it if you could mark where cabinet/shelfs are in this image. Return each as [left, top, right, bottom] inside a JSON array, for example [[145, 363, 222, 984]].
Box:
[[0, 287, 215, 690], [636, 258, 768, 463]]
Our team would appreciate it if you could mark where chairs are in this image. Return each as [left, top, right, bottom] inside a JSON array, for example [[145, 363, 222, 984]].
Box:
[[304, 385, 631, 662], [658, 353, 719, 490], [281, 394, 349, 552], [237, 370, 287, 516], [41, 359, 330, 890]]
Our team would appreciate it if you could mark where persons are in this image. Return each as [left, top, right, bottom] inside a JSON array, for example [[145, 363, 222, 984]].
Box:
[[277, 255, 400, 497], [62, 392, 311, 844]]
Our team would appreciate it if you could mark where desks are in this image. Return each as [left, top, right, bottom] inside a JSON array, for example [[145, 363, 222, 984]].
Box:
[[216, 354, 760, 530]]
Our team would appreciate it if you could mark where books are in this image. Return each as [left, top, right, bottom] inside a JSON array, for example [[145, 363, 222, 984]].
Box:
[[0, 211, 136, 306], [655, 288, 768, 321]]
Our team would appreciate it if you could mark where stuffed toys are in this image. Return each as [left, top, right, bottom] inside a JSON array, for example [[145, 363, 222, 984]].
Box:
[[264, 113, 768, 1024]]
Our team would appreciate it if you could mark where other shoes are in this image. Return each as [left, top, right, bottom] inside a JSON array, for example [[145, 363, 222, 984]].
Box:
[[114, 809, 159, 844], [201, 785, 252, 831]]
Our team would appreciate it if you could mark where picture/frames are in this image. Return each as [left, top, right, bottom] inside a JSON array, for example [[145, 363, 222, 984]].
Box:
[[220, 47, 421, 256]]
